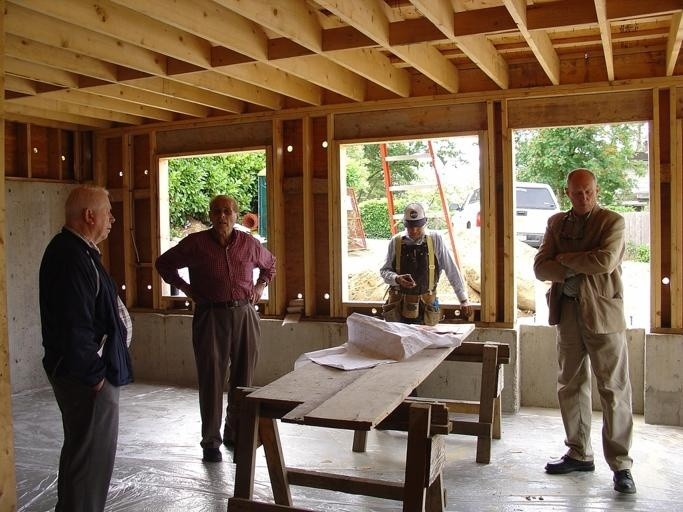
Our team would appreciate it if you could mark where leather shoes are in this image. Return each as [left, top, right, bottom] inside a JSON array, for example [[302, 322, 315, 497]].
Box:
[[543, 450, 599, 474], [612, 466, 640, 493], [200, 443, 225, 463]]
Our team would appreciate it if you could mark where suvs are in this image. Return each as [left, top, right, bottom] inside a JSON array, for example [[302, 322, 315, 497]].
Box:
[[447, 178, 565, 248]]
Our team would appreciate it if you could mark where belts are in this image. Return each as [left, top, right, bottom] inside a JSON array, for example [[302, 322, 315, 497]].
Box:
[[562, 294, 580, 302], [195, 297, 253, 309]]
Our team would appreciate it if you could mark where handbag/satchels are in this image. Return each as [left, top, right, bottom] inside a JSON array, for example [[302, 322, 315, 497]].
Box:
[[382, 291, 442, 326]]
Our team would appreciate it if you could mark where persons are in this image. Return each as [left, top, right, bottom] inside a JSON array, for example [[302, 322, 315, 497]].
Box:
[[156, 197, 276, 464], [378, 201, 472, 326], [534, 169, 635, 494], [38, 188, 134, 508]]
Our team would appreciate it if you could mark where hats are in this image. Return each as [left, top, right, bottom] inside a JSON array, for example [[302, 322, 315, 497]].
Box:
[[400, 202, 430, 227]]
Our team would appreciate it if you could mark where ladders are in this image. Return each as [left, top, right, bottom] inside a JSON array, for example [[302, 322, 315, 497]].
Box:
[[380, 141, 461, 273]]
